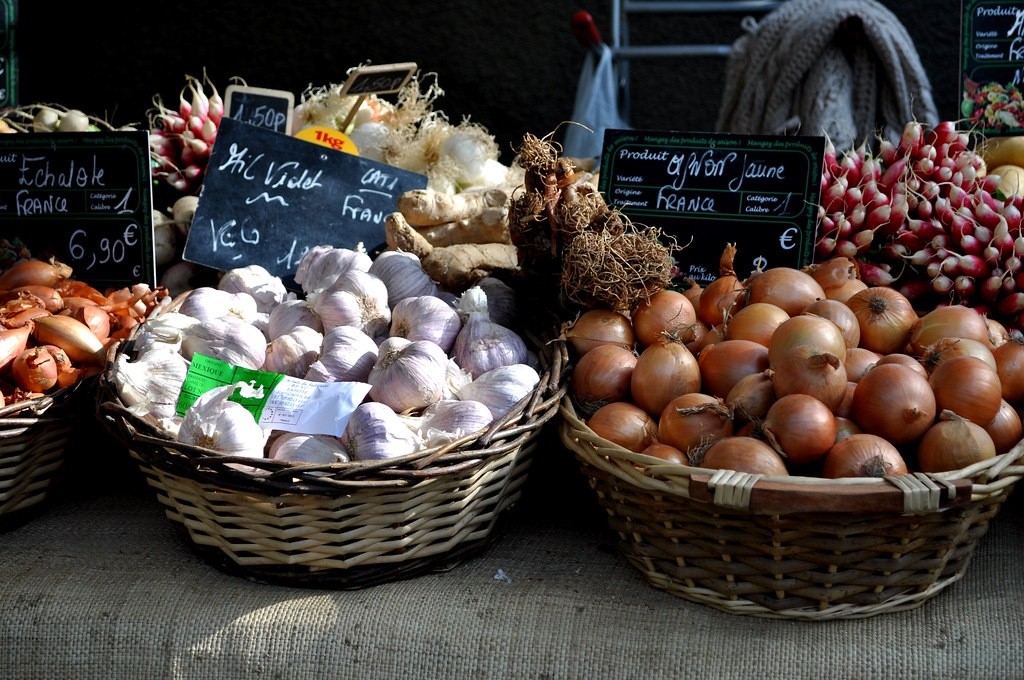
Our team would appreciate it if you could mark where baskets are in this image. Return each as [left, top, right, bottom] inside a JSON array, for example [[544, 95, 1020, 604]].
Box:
[[100, 282, 572, 596], [557, 355, 1024, 625], [0, 289, 172, 519]]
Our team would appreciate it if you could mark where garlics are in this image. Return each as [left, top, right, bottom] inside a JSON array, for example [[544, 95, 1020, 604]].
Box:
[[105, 242, 539, 469]]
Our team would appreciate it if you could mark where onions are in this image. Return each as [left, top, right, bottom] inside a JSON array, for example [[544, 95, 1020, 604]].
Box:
[[560, 258, 1024, 484]]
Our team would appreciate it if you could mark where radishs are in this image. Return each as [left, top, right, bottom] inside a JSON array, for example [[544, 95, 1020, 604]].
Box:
[[0, 102, 216, 298]]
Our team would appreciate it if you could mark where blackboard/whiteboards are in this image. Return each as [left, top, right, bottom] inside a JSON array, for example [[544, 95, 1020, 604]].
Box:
[[339, 63, 418, 97], [0, 130, 157, 297], [595, 128, 828, 290], [181, 117, 429, 299], [956, 0, 1024, 135], [223, 84, 294, 137]]
